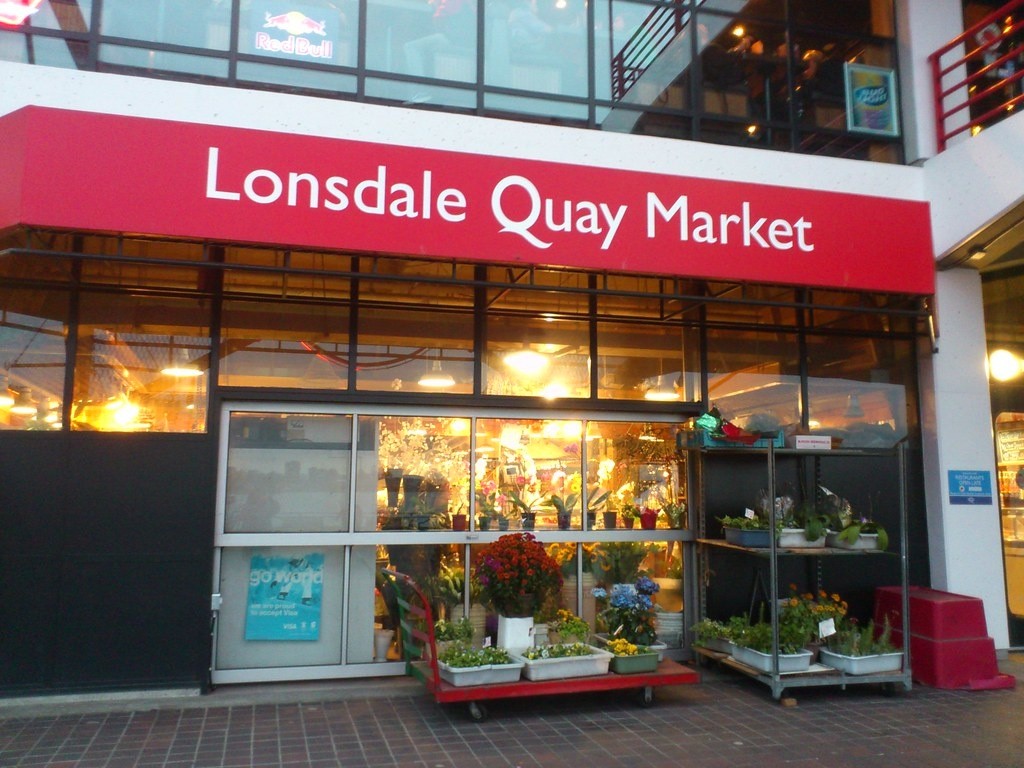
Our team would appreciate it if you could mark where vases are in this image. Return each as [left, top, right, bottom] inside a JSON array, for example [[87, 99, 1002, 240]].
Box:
[[624, 518, 635, 528], [496, 616, 535, 652], [594, 632, 667, 662], [561, 573, 596, 632], [603, 511, 617, 529], [513, 642, 613, 681], [452, 603, 487, 646], [611, 650, 658, 674], [803, 640, 822, 666]]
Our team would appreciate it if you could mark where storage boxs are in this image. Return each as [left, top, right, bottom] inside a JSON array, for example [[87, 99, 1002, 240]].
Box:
[[688, 429, 785, 448], [789, 434, 831, 449]]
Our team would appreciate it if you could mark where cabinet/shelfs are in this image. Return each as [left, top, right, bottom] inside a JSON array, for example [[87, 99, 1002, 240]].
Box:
[[691, 437, 914, 702]]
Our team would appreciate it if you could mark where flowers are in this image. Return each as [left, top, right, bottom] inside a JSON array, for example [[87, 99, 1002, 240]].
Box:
[[523, 611, 589, 658], [545, 542, 613, 573], [780, 585, 846, 642], [621, 505, 633, 517], [592, 577, 659, 645], [434, 563, 490, 606], [604, 638, 640, 655], [476, 533, 564, 618]]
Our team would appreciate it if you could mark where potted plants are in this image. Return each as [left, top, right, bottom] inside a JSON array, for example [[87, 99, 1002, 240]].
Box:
[[828, 506, 887, 550], [437, 653, 525, 686], [721, 515, 783, 547], [580, 489, 611, 530], [498, 510, 515, 531], [542, 492, 579, 529], [690, 617, 730, 654], [777, 516, 832, 548], [507, 491, 549, 531], [729, 624, 813, 672], [452, 504, 466, 531], [820, 619, 903, 674], [478, 505, 493, 531]]
